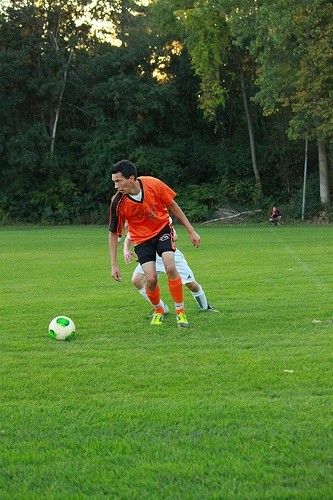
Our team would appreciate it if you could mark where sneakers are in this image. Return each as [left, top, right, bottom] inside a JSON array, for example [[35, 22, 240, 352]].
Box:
[[149, 311, 167, 326], [174, 312, 189, 328], [147, 303, 170, 319], [203, 305, 220, 313]]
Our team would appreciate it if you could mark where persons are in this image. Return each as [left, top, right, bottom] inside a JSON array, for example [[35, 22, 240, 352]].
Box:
[[269, 206, 281, 226], [124, 217, 219, 319], [107, 160, 200, 328]]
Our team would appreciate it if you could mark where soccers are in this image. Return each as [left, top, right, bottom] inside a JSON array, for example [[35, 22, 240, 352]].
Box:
[[49, 316, 76, 341]]
